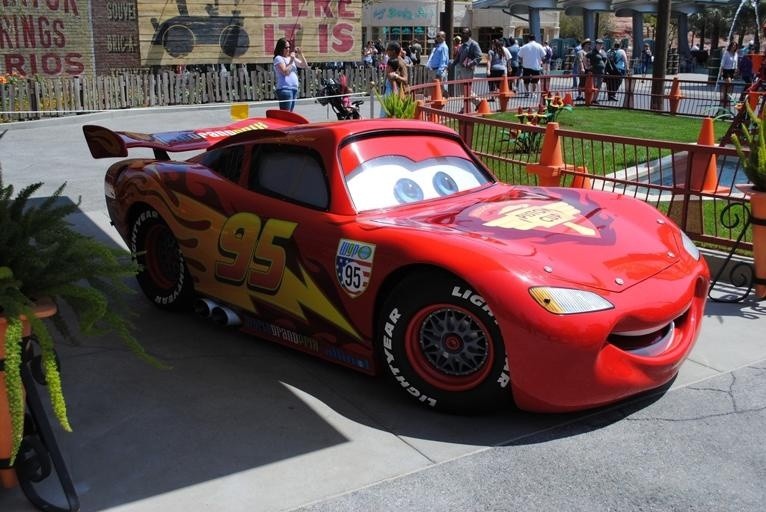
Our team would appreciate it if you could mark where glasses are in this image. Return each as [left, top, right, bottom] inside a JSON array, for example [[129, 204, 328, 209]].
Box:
[[284, 46, 290, 49]]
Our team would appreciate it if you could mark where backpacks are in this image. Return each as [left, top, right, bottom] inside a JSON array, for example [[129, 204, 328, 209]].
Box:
[[607, 48, 623, 74]]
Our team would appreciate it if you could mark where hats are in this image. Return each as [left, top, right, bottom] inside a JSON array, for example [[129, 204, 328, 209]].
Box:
[[581, 38, 592, 44], [596, 38, 604, 43]]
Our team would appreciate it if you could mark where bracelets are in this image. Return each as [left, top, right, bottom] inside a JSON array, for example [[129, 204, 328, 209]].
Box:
[[395, 73, 399, 80]]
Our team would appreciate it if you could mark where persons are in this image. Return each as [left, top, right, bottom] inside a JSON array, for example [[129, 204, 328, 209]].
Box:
[[740, 50, 756, 102], [450, 28, 484, 115], [380, 42, 409, 95], [640, 43, 652, 83], [362, 31, 631, 106], [690, 43, 700, 52], [380, 57, 401, 118], [718, 41, 740, 108], [741, 41, 756, 56], [271, 38, 308, 112]]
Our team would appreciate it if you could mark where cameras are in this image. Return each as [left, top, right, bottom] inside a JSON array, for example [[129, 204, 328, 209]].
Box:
[[294, 47, 299, 53]]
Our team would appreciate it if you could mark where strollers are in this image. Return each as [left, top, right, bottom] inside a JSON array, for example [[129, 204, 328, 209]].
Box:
[[307, 72, 364, 121]]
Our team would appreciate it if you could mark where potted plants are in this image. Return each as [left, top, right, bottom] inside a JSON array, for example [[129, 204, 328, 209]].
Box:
[[730, 98, 766, 299], [0, 127, 175, 490]]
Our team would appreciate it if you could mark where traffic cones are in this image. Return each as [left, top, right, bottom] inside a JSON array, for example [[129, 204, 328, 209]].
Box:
[[687, 118, 731, 196]]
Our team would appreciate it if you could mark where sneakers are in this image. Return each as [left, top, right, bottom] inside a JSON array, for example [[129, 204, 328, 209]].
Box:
[[571, 87, 618, 104], [487, 99, 495, 101], [457, 100, 483, 114], [525, 91, 536, 97]]
